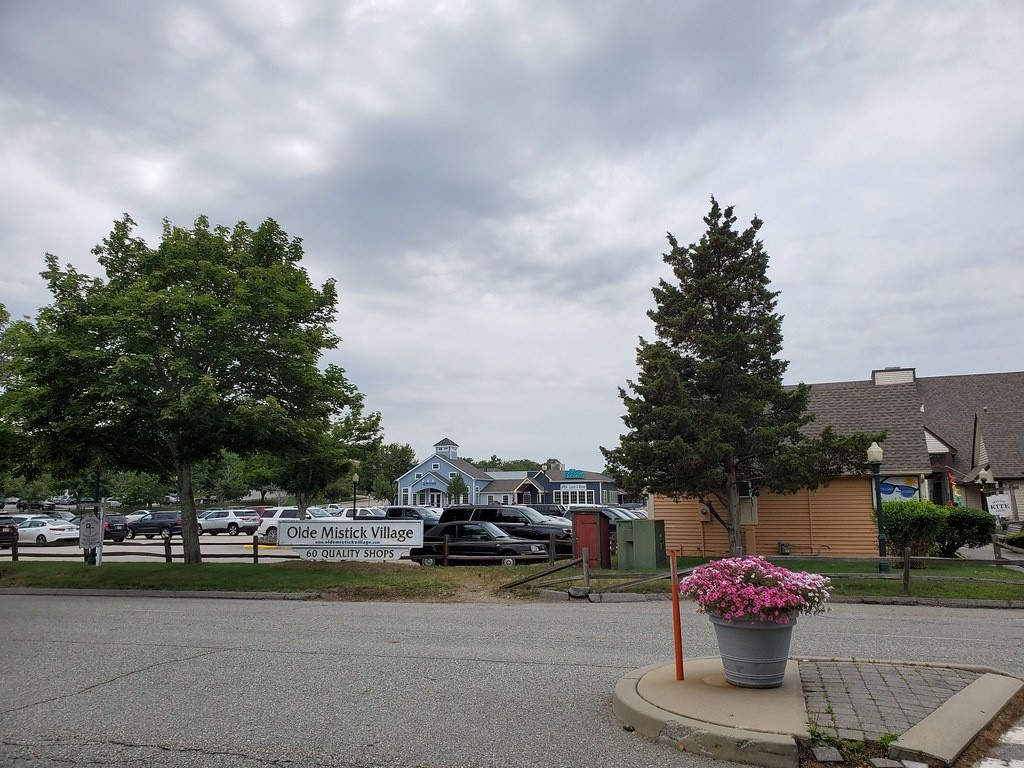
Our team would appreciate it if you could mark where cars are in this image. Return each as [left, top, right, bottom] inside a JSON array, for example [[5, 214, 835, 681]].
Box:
[[334, 508, 388, 520], [408, 520, 550, 566], [0, 511, 79, 549], [521, 504, 649, 555], [125, 509, 183, 539]]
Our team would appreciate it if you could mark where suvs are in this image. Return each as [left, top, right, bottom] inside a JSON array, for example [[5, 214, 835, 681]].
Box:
[[438, 505, 573, 553], [387, 505, 439, 521], [70, 513, 127, 543], [196, 509, 261, 536], [257, 508, 340, 539]]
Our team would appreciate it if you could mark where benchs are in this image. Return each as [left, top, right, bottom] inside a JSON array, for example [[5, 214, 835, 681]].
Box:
[[995, 523, 1021, 540]]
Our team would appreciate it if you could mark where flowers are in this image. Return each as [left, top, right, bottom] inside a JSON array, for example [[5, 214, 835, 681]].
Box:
[[677, 555, 833, 624]]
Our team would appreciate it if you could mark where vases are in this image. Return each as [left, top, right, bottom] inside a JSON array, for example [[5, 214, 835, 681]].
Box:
[[708, 607, 797, 689]]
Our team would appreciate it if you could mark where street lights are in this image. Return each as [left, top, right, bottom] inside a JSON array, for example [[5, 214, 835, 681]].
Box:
[[352, 474, 359, 520], [867, 442, 890, 573]]
